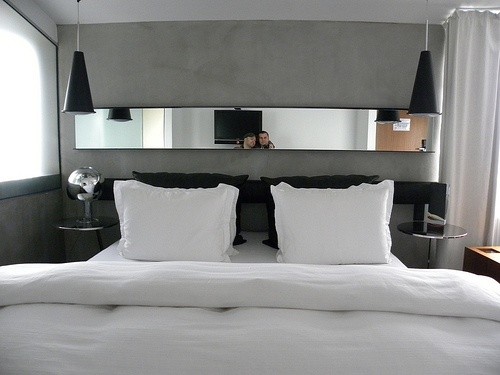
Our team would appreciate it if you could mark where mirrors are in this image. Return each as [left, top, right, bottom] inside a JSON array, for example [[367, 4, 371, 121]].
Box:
[[73, 106, 438, 153], [67, 167, 105, 223]]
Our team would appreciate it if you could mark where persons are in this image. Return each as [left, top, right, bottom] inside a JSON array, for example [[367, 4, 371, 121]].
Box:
[[233, 132, 257, 151], [256, 131, 276, 150]]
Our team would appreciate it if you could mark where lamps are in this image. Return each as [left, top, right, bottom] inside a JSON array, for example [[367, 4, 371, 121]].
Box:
[[375, 110, 402, 124], [407, 0, 438, 117], [61, 0, 97, 117], [107, 109, 133, 122]]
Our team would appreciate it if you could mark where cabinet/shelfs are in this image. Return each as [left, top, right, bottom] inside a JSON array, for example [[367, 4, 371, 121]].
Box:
[[463, 246, 500, 283]]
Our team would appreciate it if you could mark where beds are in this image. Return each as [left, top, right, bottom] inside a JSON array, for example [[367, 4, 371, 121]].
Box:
[[0, 179, 500, 375]]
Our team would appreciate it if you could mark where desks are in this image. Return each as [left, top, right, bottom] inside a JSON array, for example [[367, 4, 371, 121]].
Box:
[[397, 222, 468, 269], [58, 216, 119, 250]]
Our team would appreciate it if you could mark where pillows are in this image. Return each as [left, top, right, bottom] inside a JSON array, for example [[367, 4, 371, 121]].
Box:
[[132, 170, 249, 246], [260, 175, 380, 252], [114, 180, 240, 263], [270, 179, 394, 265]]
